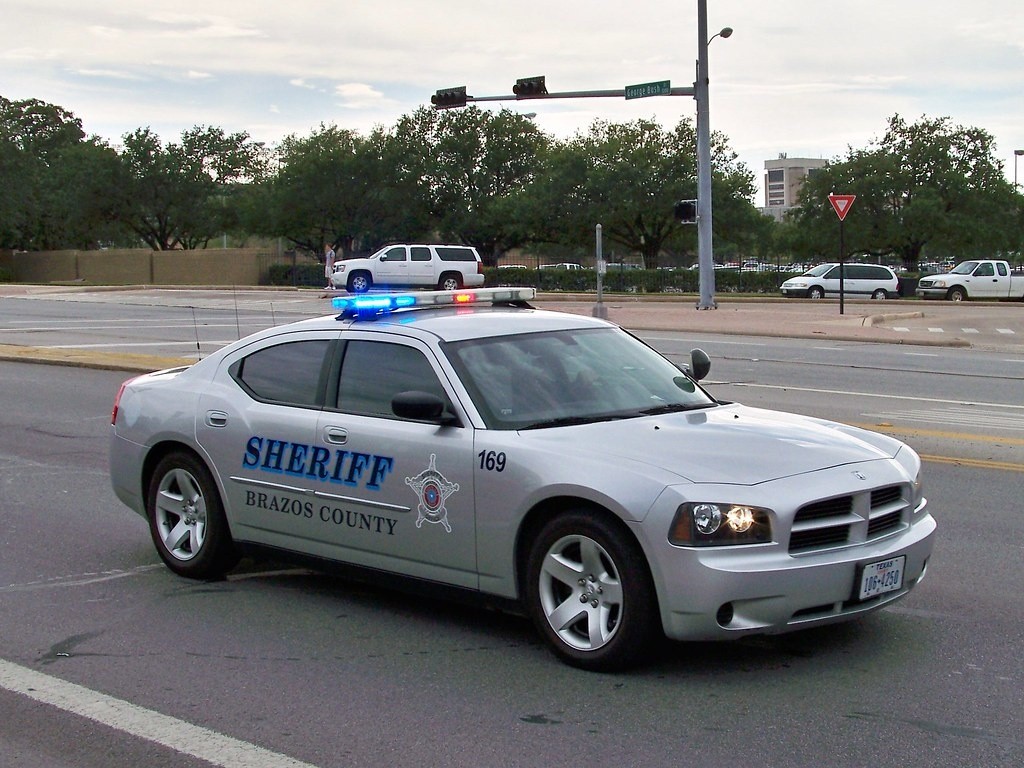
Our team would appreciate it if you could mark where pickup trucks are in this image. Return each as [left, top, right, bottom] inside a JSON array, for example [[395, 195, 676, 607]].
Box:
[[915, 260, 1024, 301]]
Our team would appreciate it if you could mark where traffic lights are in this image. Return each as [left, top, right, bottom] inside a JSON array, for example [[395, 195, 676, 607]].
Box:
[[512, 76, 545, 100], [672, 201, 691, 221], [431, 86, 466, 110]]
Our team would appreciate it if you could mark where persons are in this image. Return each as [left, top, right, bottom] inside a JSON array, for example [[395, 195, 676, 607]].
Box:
[[502, 341, 604, 414], [325, 242, 338, 290]]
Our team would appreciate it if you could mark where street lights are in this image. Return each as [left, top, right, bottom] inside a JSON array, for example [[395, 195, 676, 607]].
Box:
[[430, 1, 733, 311], [1014, 150, 1024, 195]]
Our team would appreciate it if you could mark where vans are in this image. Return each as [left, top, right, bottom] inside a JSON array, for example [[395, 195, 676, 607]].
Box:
[[779, 263, 904, 300]]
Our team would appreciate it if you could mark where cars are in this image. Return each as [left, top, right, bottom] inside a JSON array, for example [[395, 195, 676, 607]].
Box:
[[108, 287, 936, 671], [498, 258, 803, 272]]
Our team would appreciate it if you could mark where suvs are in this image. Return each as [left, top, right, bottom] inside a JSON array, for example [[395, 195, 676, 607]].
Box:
[[329, 244, 485, 291]]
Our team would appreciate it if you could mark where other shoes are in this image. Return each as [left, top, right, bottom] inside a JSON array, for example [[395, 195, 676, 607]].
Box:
[[332, 287, 336, 290], [324, 286, 331, 290]]
